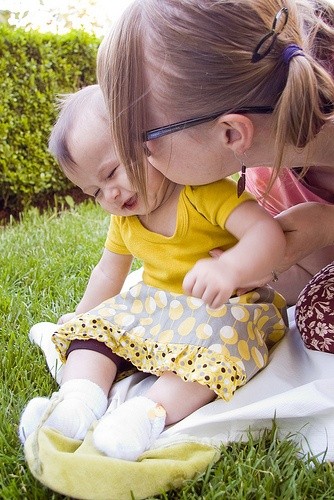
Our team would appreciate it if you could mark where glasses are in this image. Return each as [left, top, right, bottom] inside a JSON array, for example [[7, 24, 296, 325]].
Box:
[[130, 105, 278, 157]]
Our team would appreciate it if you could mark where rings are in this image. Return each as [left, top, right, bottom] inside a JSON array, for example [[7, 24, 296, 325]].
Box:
[[272, 271, 279, 284]]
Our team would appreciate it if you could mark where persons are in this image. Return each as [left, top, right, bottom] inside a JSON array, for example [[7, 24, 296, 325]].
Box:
[[97, 0, 334, 355], [42, 84, 289, 462]]
